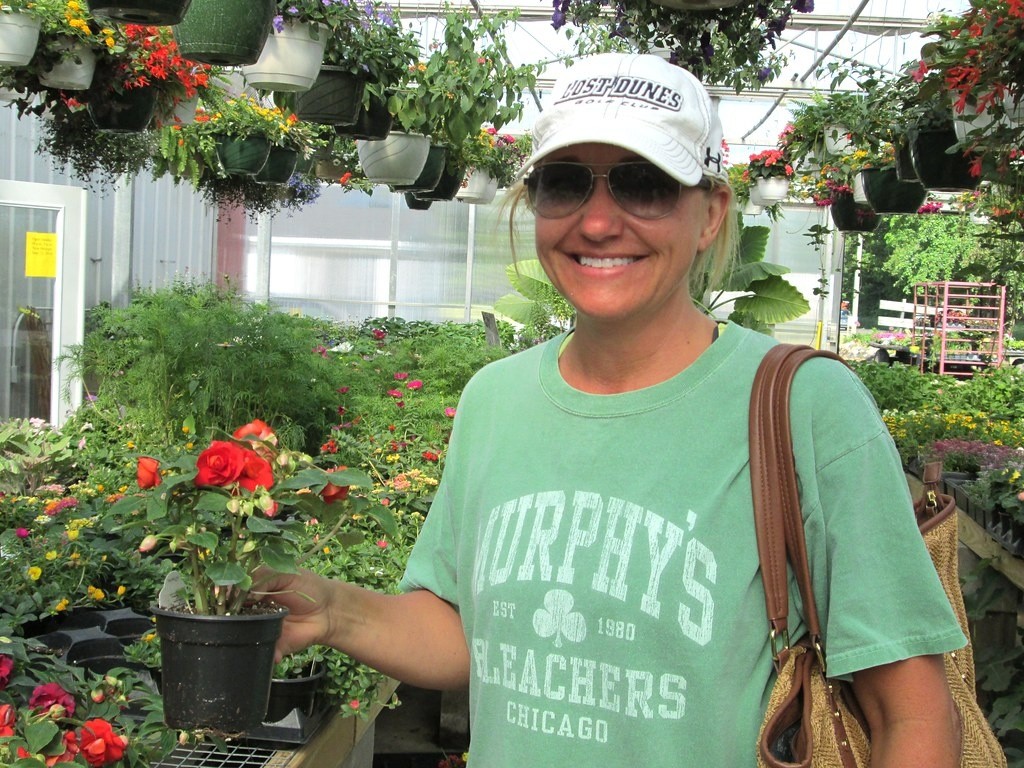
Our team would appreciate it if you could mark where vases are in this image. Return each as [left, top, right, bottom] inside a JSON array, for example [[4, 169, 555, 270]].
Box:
[[38, 35, 98, 90], [88, 74, 159, 132], [827, 188, 882, 231], [414, 161, 467, 201], [895, 139, 921, 182], [911, 129, 982, 192], [294, 64, 366, 126], [750, 188, 777, 206], [252, 146, 298, 184], [454, 167, 500, 204], [164, 88, 199, 125], [88, 1, 189, 26], [853, 172, 868, 203], [405, 192, 432, 210], [823, 124, 857, 155], [950, 88, 1005, 141], [245, 20, 331, 93], [156, 599, 290, 732], [0, 13, 43, 65], [312, 155, 346, 180], [861, 167, 924, 216], [213, 131, 272, 175], [389, 146, 447, 192], [335, 103, 393, 140], [756, 176, 789, 200], [175, 1, 275, 68], [355, 130, 433, 185]]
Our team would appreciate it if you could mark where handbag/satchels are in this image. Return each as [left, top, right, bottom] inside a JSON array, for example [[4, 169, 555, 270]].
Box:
[[748, 344, 1010, 768]]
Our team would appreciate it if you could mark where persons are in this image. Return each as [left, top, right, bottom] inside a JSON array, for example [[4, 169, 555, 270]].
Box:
[[249, 54, 968, 768]]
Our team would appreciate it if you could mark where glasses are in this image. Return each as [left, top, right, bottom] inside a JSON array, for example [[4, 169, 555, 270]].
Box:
[[524, 160, 708, 220]]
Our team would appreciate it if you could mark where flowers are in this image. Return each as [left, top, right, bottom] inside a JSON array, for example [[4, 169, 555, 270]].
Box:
[[0, 1, 1023, 217], [103, 419, 403, 616]]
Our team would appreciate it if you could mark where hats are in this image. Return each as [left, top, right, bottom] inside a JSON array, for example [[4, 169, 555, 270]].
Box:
[[514, 53, 730, 186]]
